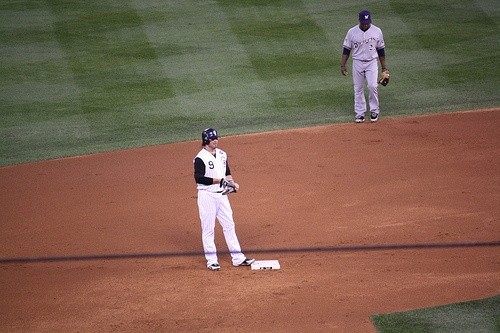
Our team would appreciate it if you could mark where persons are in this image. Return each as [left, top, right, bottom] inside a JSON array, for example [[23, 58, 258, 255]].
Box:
[[193, 128, 255, 270], [341, 10, 390, 122]]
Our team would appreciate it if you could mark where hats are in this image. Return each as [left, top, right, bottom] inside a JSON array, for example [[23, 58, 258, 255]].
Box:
[[359, 10, 372, 23]]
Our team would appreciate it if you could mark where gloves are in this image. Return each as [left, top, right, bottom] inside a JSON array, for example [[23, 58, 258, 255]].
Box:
[[220, 178, 239, 195]]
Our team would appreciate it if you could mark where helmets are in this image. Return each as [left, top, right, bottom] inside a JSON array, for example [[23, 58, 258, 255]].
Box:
[[202, 128, 218, 143]]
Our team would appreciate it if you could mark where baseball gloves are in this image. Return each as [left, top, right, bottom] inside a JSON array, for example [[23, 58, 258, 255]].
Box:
[[220, 179, 240, 195], [379, 70, 390, 86]]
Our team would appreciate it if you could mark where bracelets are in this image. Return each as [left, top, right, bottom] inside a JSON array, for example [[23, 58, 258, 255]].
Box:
[[382, 67, 386, 69]]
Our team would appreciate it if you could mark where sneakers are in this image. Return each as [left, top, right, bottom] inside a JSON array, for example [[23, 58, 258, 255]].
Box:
[[208, 264, 220, 270], [370, 112, 378, 122], [354, 116, 365, 122], [239, 258, 255, 265]]
